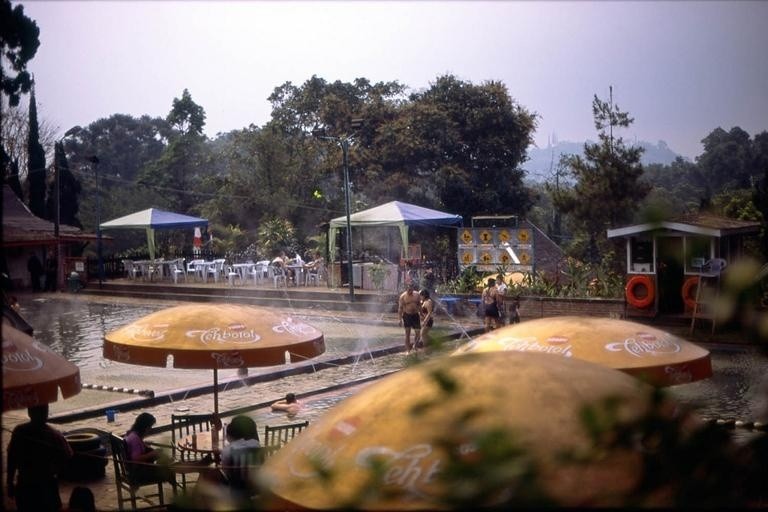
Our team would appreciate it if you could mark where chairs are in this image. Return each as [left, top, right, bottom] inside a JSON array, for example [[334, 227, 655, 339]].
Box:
[[263, 419, 309, 455], [168, 413, 216, 492], [691, 257, 729, 338], [122, 253, 325, 288], [221, 441, 282, 505], [103, 430, 178, 512]]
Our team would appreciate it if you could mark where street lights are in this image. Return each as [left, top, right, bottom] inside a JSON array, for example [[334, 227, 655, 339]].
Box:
[[85, 154, 105, 289], [315, 130, 364, 304]]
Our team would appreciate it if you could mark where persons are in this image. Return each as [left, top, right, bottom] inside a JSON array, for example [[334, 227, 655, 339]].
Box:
[[398, 285, 422, 355], [418, 288, 434, 347], [271, 248, 322, 287], [271, 393, 302, 415], [67, 486, 96, 511], [28, 248, 84, 293], [220, 414, 265, 467], [6, 404, 76, 511], [483, 280, 498, 331], [497, 275, 507, 304], [123, 412, 174, 487]]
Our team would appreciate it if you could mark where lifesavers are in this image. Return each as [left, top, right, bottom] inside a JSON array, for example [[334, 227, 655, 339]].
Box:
[[625, 276, 654, 308], [683, 277, 710, 309]]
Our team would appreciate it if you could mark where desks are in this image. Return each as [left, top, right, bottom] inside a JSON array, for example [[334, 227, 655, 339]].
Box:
[[436, 296, 462, 321], [467, 299, 485, 319], [176, 425, 286, 467], [345, 260, 399, 292]]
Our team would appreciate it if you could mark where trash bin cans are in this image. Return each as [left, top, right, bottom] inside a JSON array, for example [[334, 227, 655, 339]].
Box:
[[66, 272, 80, 294]]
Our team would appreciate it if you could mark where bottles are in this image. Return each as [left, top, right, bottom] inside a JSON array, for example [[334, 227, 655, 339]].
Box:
[[191, 431, 197, 447], [222, 423, 227, 441]]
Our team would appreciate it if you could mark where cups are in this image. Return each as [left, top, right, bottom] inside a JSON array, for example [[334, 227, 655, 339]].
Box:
[[106, 410, 115, 422]]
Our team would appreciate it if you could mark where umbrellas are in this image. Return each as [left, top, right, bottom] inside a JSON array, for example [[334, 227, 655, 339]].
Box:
[[453, 313, 713, 386], [103, 302, 326, 438], [1, 322, 82, 412], [251, 350, 705, 511]]
